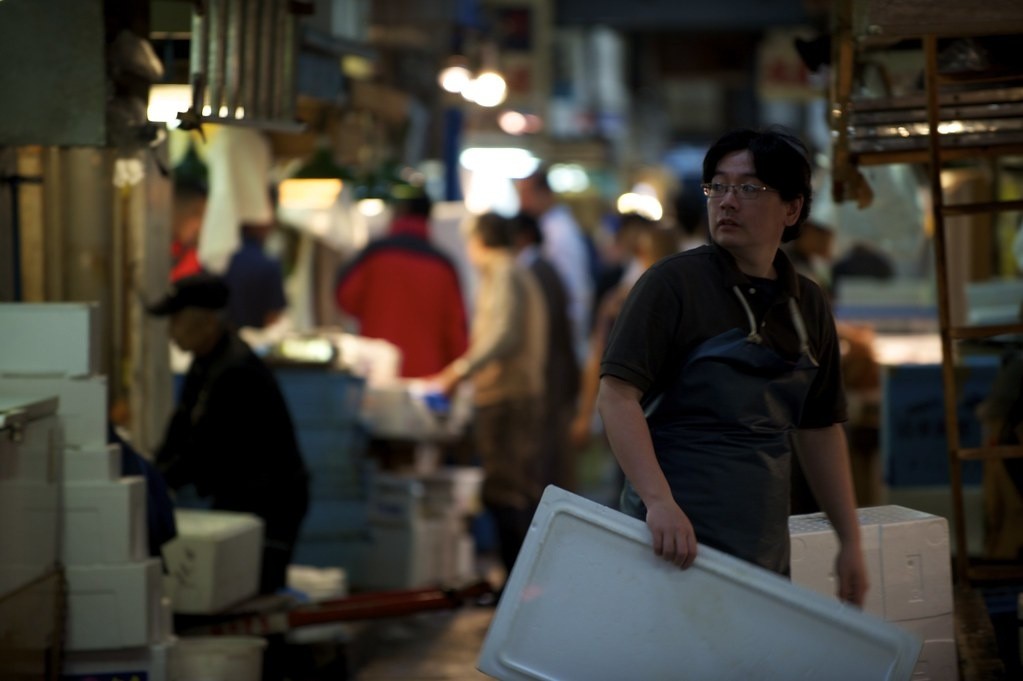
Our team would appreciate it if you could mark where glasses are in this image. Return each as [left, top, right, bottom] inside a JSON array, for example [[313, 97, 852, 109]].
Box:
[[701, 183, 780, 200]]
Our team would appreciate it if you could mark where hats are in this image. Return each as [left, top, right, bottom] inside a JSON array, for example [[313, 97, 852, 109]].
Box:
[[146, 277, 227, 317]]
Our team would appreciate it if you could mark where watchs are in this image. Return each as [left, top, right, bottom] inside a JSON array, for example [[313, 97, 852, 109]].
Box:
[[450, 357, 471, 378]]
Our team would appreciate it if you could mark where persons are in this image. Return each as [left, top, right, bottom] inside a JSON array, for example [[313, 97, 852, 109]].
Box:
[[329, 182, 471, 475], [106, 269, 308, 634], [594, 126, 873, 608], [223, 221, 290, 331], [418, 133, 899, 601]]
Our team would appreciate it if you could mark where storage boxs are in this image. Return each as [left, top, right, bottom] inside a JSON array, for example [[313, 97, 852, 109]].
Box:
[[0, 301, 161, 651], [787, 504, 960, 681], [0, 394, 59, 601], [0, 570, 62, 674], [161, 507, 265, 615], [268, 337, 496, 594], [878, 348, 1002, 561]]
